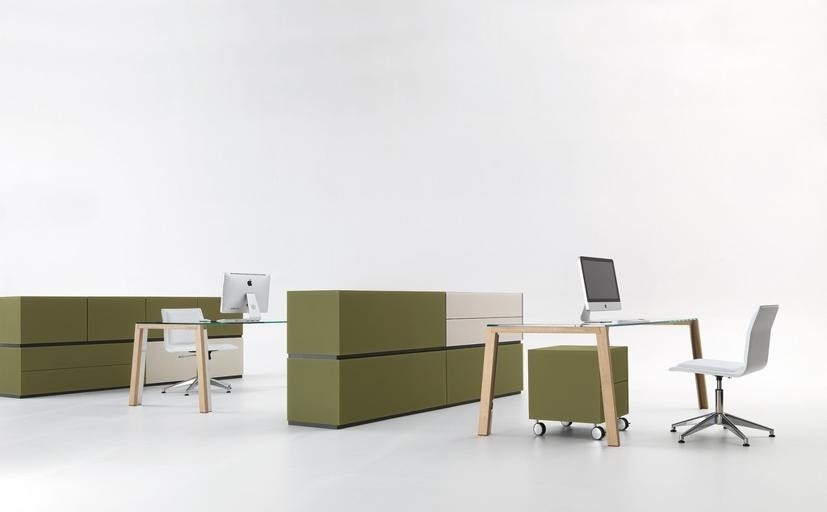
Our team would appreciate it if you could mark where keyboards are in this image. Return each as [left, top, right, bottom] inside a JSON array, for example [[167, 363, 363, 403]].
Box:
[[617, 320, 649, 323], [216, 319, 245, 323]]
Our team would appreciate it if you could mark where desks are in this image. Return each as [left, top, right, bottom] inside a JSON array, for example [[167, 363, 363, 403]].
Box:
[[128, 320, 286, 413], [478, 318, 708, 447]]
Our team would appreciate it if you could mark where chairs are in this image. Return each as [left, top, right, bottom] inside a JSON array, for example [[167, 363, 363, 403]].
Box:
[[668, 305, 779, 446], [161, 308, 233, 397]]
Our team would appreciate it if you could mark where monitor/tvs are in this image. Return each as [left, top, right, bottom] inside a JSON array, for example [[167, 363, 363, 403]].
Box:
[[577, 256, 622, 323], [220, 271, 271, 321]]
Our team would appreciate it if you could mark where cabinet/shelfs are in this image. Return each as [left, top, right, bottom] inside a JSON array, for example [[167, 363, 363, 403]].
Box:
[[527, 345, 628, 424]]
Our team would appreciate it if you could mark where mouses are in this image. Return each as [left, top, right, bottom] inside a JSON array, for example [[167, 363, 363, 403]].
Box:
[[637, 318, 645, 320], [200, 319, 211, 322]]
